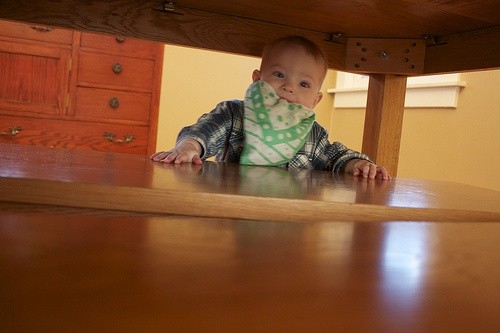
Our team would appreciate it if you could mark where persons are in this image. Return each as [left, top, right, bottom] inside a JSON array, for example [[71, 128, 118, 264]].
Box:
[[148, 33, 391, 182]]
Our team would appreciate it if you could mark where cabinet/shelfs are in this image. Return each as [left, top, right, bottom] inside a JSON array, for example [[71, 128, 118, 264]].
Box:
[[0, 0, 500, 333], [0, 20, 164, 155]]
